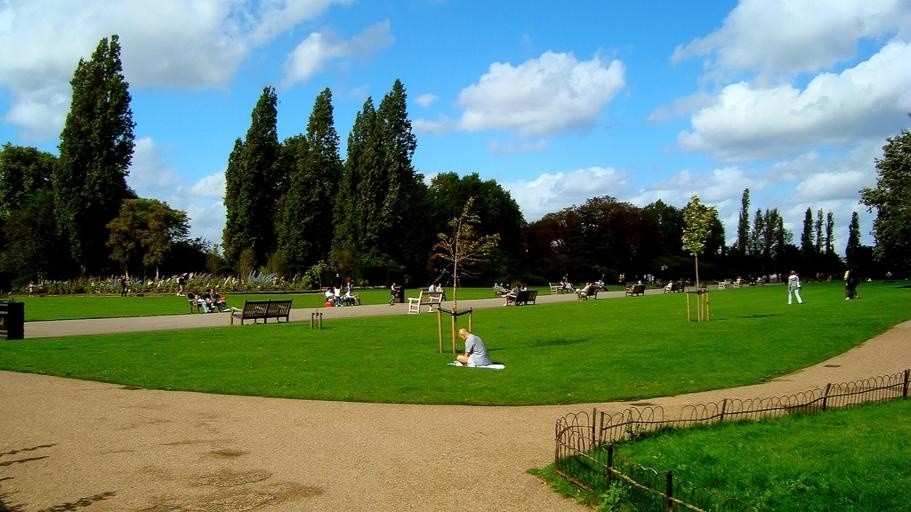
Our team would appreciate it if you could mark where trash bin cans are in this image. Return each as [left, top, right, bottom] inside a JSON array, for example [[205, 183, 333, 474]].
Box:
[[0, 301, 24, 339], [394, 287, 404, 303]]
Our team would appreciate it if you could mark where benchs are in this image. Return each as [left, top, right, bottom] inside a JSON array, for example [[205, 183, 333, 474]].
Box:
[[625, 281, 645, 297], [324, 291, 360, 306], [407, 287, 446, 313], [506, 290, 537, 306], [575, 286, 599, 300], [664, 283, 680, 294], [549, 282, 565, 294], [185, 292, 223, 313], [230, 298, 294, 325]]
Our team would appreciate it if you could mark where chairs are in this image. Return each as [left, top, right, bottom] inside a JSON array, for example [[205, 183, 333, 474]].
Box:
[[719, 278, 741, 288]]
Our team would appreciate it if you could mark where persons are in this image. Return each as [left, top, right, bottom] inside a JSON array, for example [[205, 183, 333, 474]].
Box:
[[121, 276, 132, 296], [885, 271, 893, 282], [494, 282, 528, 306], [816, 272, 832, 282], [637, 280, 643, 286], [188, 288, 227, 313], [560, 272, 608, 298], [325, 273, 357, 306], [177, 275, 186, 297], [429, 282, 437, 292], [663, 280, 673, 293], [618, 272, 625, 286], [29, 281, 34, 297], [458, 328, 493, 368], [436, 282, 443, 292], [748, 272, 783, 286], [844, 266, 860, 300], [787, 270, 803, 304], [389, 283, 397, 305]]
